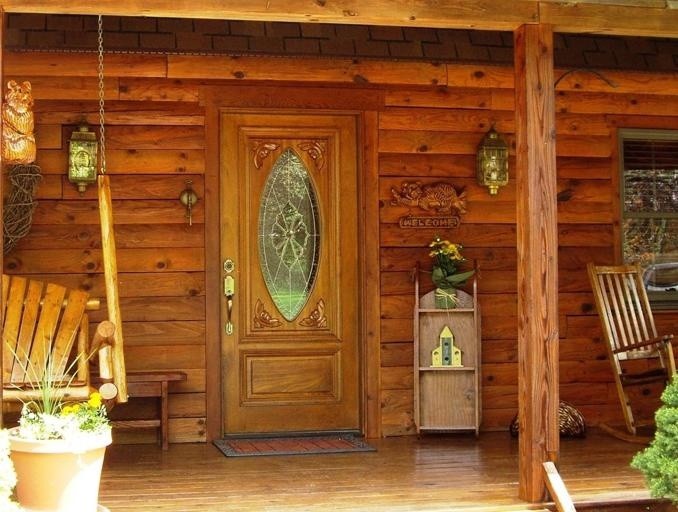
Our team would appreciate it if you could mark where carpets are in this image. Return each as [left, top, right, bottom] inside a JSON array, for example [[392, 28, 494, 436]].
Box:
[[211, 431, 381, 460]]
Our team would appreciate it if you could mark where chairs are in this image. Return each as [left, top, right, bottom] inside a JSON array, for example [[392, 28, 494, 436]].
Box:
[[585, 260, 677, 445]]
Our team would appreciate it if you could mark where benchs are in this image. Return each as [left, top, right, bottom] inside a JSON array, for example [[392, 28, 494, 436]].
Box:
[[3, 371, 188, 454]]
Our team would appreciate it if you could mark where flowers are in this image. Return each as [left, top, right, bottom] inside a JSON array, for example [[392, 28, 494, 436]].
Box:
[[426, 239, 464, 276], [1, 318, 111, 440]]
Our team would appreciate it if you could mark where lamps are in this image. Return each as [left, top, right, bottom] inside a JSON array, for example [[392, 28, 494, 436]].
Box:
[[65, 111, 99, 195], [475, 112, 510, 198]]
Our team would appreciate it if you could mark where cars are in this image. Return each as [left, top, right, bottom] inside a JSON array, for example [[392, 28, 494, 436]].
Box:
[[642, 261, 678, 292]]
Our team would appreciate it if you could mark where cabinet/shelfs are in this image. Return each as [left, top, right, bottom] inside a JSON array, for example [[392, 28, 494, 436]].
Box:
[[413, 261, 483, 440]]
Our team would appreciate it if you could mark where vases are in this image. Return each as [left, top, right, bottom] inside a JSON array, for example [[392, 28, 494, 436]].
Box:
[[3, 426, 111, 509], [434, 278, 459, 309]]
